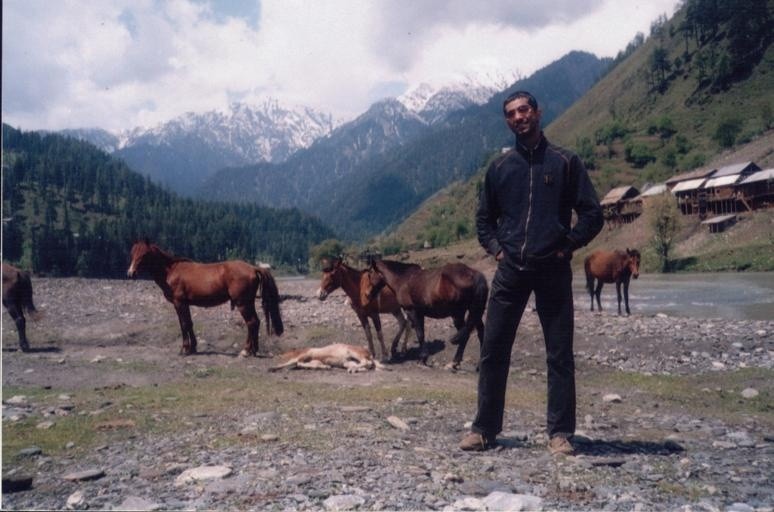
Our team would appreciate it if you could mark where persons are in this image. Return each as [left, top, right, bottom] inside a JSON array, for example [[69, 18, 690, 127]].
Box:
[[455, 89, 606, 458]]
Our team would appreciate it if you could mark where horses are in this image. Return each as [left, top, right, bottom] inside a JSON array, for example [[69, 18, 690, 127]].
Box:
[[1, 262, 38, 353], [584, 247, 641, 315], [268, 343, 392, 375], [127, 236, 283, 359], [317, 252, 412, 363], [365, 253, 489, 370]]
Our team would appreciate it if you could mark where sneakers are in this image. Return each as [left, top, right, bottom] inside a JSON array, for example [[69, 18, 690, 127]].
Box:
[[457, 429, 499, 453], [546, 431, 581, 456]]
